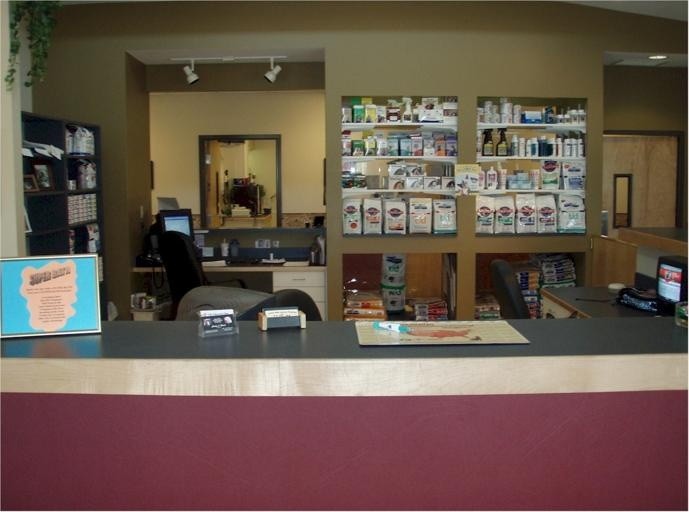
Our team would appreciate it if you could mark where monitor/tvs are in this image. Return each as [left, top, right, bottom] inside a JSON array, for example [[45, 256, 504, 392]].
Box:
[[655, 255, 688, 316], [229, 184, 262, 215], [151, 208, 196, 243]]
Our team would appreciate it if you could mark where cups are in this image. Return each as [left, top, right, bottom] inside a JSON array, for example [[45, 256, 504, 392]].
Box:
[[220, 243, 229, 257]]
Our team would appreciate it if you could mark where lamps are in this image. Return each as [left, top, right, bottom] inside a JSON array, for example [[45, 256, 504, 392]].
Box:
[[264, 65, 281, 83], [184, 65, 199, 84]]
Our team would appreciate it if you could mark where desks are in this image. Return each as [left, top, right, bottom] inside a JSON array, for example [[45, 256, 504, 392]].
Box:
[[0, 318, 689, 512], [134, 259, 327, 324]]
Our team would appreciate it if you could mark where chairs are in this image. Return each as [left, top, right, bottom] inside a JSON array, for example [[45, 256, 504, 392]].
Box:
[[178, 286, 273, 320], [239, 288, 322, 322], [491, 260, 531, 321], [163, 230, 247, 315]]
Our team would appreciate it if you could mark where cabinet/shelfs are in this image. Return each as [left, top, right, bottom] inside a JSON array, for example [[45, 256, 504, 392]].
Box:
[[22, 110, 108, 323], [343, 95, 592, 320], [539, 223, 687, 317]]
